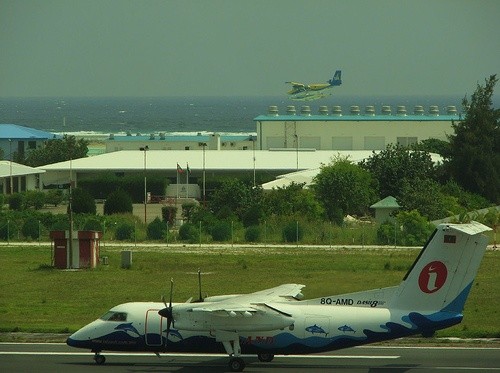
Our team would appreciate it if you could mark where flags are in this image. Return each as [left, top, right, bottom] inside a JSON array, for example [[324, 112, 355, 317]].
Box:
[[187, 164, 191, 174], [177, 164, 184, 175]]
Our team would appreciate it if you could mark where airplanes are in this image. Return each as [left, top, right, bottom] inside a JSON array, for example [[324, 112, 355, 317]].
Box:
[[281, 70, 342, 103], [64, 220, 494, 373]]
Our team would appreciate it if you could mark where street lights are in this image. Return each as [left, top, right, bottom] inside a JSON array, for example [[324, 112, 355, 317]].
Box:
[[139, 145, 149, 225], [249, 138, 255, 187], [198, 141, 207, 209], [295, 134, 298, 172]]
